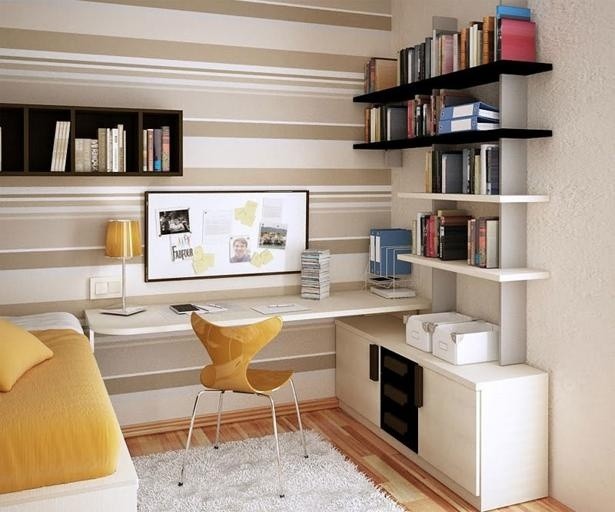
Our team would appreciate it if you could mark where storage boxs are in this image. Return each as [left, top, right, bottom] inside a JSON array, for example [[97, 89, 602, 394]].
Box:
[[405, 311, 500, 364]]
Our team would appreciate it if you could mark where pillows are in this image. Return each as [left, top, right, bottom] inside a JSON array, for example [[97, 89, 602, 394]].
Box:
[[0, 319, 54, 392]]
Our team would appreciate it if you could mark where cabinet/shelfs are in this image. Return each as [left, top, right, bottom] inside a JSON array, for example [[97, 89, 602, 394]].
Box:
[[0, 102, 183, 176], [353, 59, 553, 150], [397, 193, 550, 283]]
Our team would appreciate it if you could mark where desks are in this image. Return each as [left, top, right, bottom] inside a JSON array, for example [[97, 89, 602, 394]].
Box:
[[335, 313, 549, 512]]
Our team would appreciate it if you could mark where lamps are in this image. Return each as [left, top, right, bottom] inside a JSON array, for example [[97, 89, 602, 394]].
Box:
[[100, 219, 147, 316]]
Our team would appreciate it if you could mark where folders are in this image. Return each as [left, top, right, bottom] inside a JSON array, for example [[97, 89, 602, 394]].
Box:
[[370, 228, 412, 275], [438, 101, 499, 134]]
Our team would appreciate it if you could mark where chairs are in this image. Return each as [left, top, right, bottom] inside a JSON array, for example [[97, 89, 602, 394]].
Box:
[[178, 312, 308, 498]]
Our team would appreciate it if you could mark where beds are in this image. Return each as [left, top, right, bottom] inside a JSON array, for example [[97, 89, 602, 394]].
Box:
[[0, 312, 139, 512]]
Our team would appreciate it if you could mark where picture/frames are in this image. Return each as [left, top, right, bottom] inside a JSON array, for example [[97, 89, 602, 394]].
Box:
[[144, 190, 309, 282]]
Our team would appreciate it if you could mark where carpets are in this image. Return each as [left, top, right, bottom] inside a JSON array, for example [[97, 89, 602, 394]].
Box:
[[132, 429, 410, 512]]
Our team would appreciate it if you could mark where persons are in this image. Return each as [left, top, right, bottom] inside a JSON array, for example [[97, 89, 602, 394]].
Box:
[[231, 239, 252, 263], [171, 218, 185, 232]]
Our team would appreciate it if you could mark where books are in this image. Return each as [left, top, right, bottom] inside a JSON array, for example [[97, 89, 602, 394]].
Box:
[[301, 249, 330, 299], [467, 215, 499, 269], [51, 120, 171, 174], [364, 88, 479, 143], [369, 228, 407, 274], [412, 209, 472, 261], [370, 285, 417, 299], [362, 6, 537, 95], [375, 230, 412, 276], [425, 143, 499, 196]]
